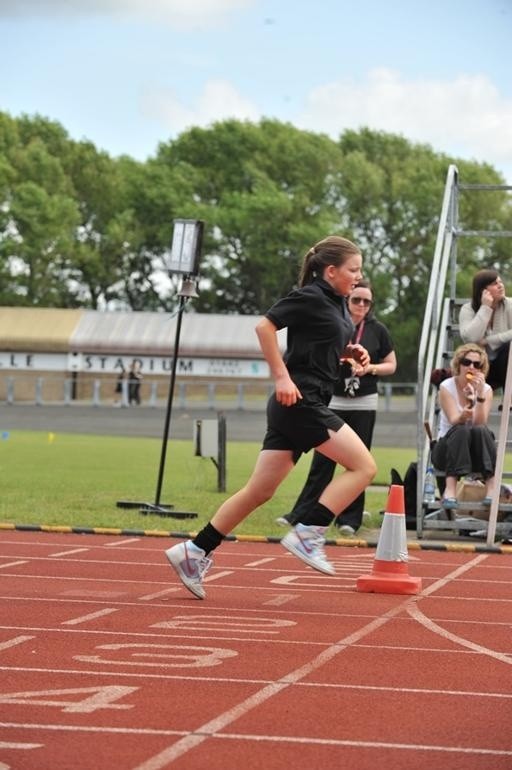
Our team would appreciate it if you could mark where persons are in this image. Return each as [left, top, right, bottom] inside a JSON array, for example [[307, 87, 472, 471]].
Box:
[[428, 268, 512, 393], [117, 363, 143, 406], [274, 278, 400, 539], [432, 343, 500, 511], [163, 233, 380, 601]]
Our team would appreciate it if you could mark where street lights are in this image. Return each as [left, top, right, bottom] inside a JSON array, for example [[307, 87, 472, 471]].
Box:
[[155, 217, 204, 509]]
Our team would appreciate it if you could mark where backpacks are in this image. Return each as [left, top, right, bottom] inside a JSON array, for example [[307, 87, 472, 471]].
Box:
[[390, 463, 419, 530]]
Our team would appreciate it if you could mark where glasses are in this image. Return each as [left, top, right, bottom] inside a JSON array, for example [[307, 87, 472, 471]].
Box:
[[459, 359, 483, 369], [350, 295, 373, 308]]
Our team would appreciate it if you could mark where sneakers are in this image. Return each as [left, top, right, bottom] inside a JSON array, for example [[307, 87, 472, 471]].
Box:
[[274, 516, 290, 526], [482, 496, 491, 506], [279, 522, 337, 577], [163, 539, 215, 600], [338, 524, 355, 536], [441, 492, 458, 509]]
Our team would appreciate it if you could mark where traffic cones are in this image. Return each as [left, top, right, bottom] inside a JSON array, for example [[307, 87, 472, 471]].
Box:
[[357, 483, 422, 595]]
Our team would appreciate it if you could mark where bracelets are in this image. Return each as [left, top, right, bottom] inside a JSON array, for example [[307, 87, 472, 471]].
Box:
[[476, 396, 486, 403]]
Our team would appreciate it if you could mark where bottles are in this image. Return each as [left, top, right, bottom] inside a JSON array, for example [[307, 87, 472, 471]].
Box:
[[423, 468, 436, 503]]
